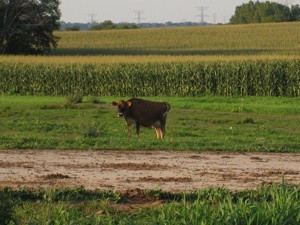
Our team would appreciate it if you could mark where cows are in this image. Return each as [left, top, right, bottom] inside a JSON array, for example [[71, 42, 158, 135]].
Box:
[[111, 97, 170, 140]]
